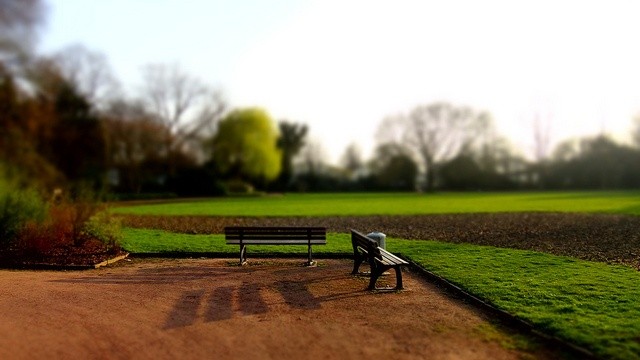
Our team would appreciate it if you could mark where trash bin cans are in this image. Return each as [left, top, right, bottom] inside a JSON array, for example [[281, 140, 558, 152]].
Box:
[[367, 231, 386, 250]]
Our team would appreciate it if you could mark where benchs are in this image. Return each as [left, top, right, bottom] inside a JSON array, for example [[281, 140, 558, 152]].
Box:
[[351, 229, 409, 292], [223, 225, 327, 268]]
